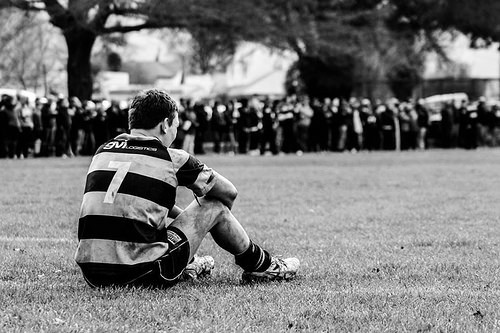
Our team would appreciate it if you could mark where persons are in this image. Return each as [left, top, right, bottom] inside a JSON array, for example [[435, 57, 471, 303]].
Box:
[[0, 92, 499, 156], [74, 88, 301, 290]]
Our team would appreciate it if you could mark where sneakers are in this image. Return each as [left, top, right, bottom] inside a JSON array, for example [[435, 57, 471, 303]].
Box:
[[242, 257, 301, 283], [184, 255, 215, 278]]
